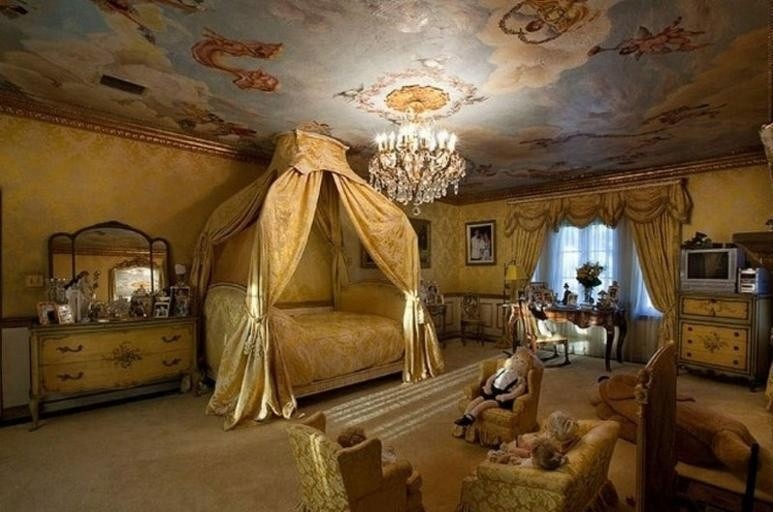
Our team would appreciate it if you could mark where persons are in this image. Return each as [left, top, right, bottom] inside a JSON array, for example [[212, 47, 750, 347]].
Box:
[[500, 411, 581, 457], [487, 437, 569, 470], [454, 347, 536, 427]]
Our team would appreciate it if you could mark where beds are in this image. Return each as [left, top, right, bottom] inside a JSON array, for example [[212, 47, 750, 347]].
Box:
[[199, 281, 430, 399]]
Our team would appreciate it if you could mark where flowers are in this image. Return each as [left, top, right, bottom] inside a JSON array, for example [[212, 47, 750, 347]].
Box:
[[575, 261, 604, 288]]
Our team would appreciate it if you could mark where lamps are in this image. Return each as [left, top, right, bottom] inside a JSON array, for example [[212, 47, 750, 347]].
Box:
[[366, 103, 468, 216], [505, 263, 528, 304]]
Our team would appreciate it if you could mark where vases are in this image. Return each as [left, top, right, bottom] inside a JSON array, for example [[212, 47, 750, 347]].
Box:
[[582, 285, 594, 309]]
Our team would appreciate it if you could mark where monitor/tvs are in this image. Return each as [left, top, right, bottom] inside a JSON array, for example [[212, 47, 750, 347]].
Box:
[[679, 248, 738, 292]]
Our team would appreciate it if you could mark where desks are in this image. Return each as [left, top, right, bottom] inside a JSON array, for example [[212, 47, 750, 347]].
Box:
[[503, 300, 628, 373]]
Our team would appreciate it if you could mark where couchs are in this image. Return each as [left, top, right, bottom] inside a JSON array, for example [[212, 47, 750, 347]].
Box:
[[459, 419, 620, 512]]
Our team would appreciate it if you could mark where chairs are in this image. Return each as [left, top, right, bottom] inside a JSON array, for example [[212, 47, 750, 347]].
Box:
[[454, 354, 545, 447], [635, 339, 773, 512], [508, 301, 572, 367], [458, 291, 487, 348], [286, 409, 423, 512], [423, 279, 447, 349]]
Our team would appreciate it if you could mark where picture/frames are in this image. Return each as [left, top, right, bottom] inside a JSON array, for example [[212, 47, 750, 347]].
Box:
[[464, 219, 497, 267], [359, 218, 433, 270]]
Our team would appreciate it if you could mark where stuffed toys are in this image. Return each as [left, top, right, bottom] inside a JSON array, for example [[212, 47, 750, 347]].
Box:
[[590, 373, 758, 472], [337, 425, 398, 464], [528, 291, 555, 337]]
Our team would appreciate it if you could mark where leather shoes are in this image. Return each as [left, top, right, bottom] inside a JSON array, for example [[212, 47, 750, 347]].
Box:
[[455, 414, 476, 427]]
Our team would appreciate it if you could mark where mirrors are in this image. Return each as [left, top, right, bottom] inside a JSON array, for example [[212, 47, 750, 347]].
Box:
[[45, 220, 174, 320]]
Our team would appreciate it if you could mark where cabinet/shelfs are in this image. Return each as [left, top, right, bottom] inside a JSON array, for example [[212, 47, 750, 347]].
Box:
[[26, 315, 202, 433], [673, 288, 773, 393]]
